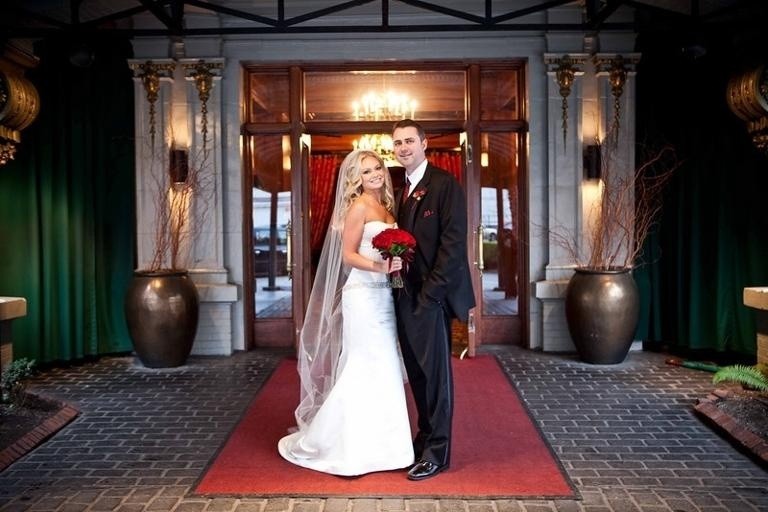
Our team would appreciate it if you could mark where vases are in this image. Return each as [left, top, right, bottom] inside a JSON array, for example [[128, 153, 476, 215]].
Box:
[[124, 270, 199, 368], [565, 266, 641, 364]]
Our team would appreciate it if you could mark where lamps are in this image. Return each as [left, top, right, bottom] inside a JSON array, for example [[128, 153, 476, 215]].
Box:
[[351, 71, 416, 167], [170, 150, 187, 183], [582, 144, 602, 177]]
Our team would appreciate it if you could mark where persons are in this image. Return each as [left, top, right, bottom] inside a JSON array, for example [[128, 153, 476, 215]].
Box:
[[393, 120, 477, 479], [277, 149, 416, 479]]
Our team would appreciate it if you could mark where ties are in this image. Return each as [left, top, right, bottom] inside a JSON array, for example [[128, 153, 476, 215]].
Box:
[[402, 178, 410, 204]]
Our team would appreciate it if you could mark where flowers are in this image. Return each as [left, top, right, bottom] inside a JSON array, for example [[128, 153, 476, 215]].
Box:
[[372, 228, 416, 289]]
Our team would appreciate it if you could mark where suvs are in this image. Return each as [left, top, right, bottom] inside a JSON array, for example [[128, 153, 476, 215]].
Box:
[[254, 224, 288, 277]]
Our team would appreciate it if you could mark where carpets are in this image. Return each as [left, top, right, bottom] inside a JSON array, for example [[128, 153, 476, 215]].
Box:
[[182, 356, 583, 501]]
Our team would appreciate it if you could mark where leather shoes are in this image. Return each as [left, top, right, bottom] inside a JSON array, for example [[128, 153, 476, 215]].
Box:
[[407, 460, 448, 480]]
